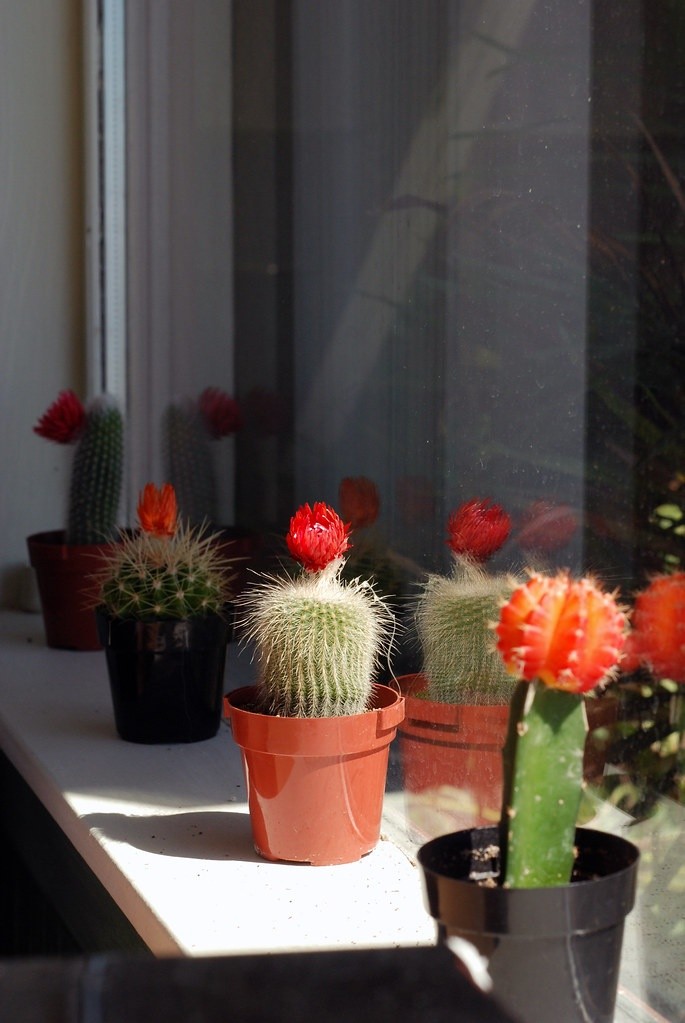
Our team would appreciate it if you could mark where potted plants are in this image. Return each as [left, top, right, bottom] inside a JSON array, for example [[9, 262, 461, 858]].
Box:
[[635, 570, 685, 1023], [164, 401, 250, 642], [391, 550, 518, 835], [412, 566, 640, 1023], [224, 556, 406, 866], [84, 513, 235, 746], [27, 392, 138, 653], [336, 543, 407, 685]]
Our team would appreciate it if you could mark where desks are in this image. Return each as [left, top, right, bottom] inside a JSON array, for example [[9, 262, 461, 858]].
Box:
[[0, 600, 685, 1023]]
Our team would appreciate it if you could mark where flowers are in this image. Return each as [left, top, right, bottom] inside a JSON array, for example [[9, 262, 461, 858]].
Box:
[[134, 481, 178, 537], [32, 388, 84, 444], [339, 475, 381, 528], [515, 499, 582, 555], [444, 496, 511, 563], [285, 501, 353, 575], [200, 384, 241, 441]]
